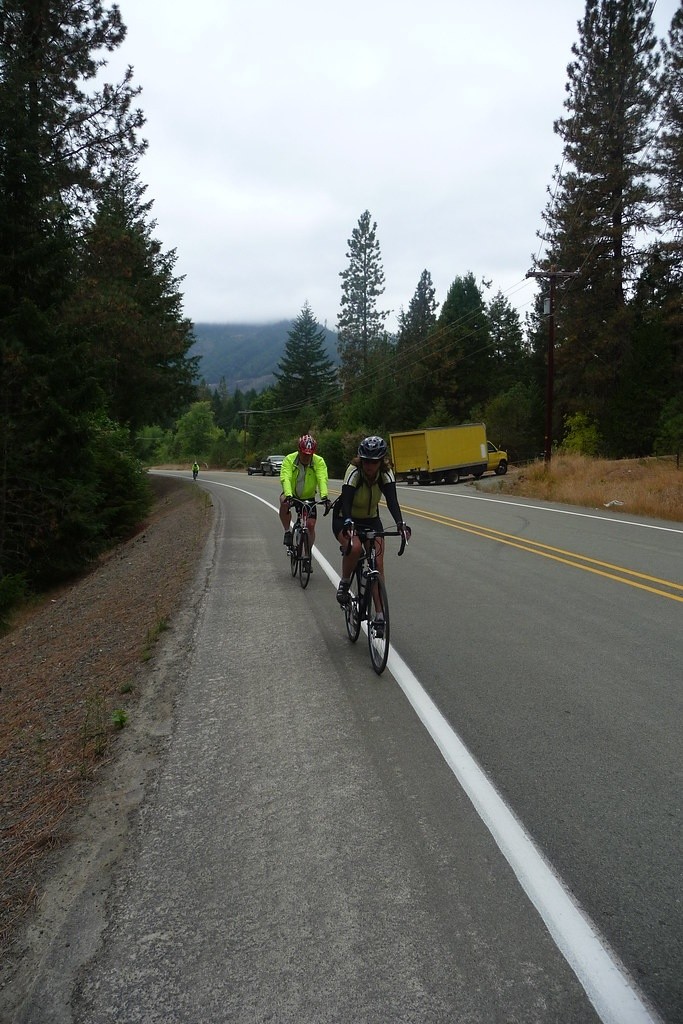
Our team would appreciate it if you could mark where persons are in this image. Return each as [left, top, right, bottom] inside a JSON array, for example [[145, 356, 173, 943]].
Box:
[[192, 462, 199, 478], [332, 436, 411, 636], [279, 435, 332, 573]]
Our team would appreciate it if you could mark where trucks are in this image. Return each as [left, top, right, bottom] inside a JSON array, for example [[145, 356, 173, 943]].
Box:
[[389, 423, 510, 484]]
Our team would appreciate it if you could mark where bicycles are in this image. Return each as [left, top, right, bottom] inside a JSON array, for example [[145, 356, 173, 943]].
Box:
[[285, 495, 333, 588], [341, 519, 408, 673], [193, 471, 198, 481]]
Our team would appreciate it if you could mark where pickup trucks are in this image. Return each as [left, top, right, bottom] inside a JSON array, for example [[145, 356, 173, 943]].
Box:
[[260, 455, 286, 476]]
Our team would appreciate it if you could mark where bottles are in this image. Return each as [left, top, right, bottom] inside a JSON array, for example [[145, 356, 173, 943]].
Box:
[[360, 571, 369, 596]]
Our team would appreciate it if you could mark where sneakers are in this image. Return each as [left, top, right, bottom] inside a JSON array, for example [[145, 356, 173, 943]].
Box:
[[373, 617, 386, 638], [283, 531, 293, 546], [336, 579, 350, 605], [303, 559, 313, 573]]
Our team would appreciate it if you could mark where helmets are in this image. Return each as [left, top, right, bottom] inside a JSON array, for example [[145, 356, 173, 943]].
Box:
[[297, 434, 318, 455], [194, 462, 197, 464], [358, 435, 388, 459]]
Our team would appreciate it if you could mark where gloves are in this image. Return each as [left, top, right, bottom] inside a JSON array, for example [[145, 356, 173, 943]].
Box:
[[284, 497, 294, 506], [322, 499, 330, 517]]
